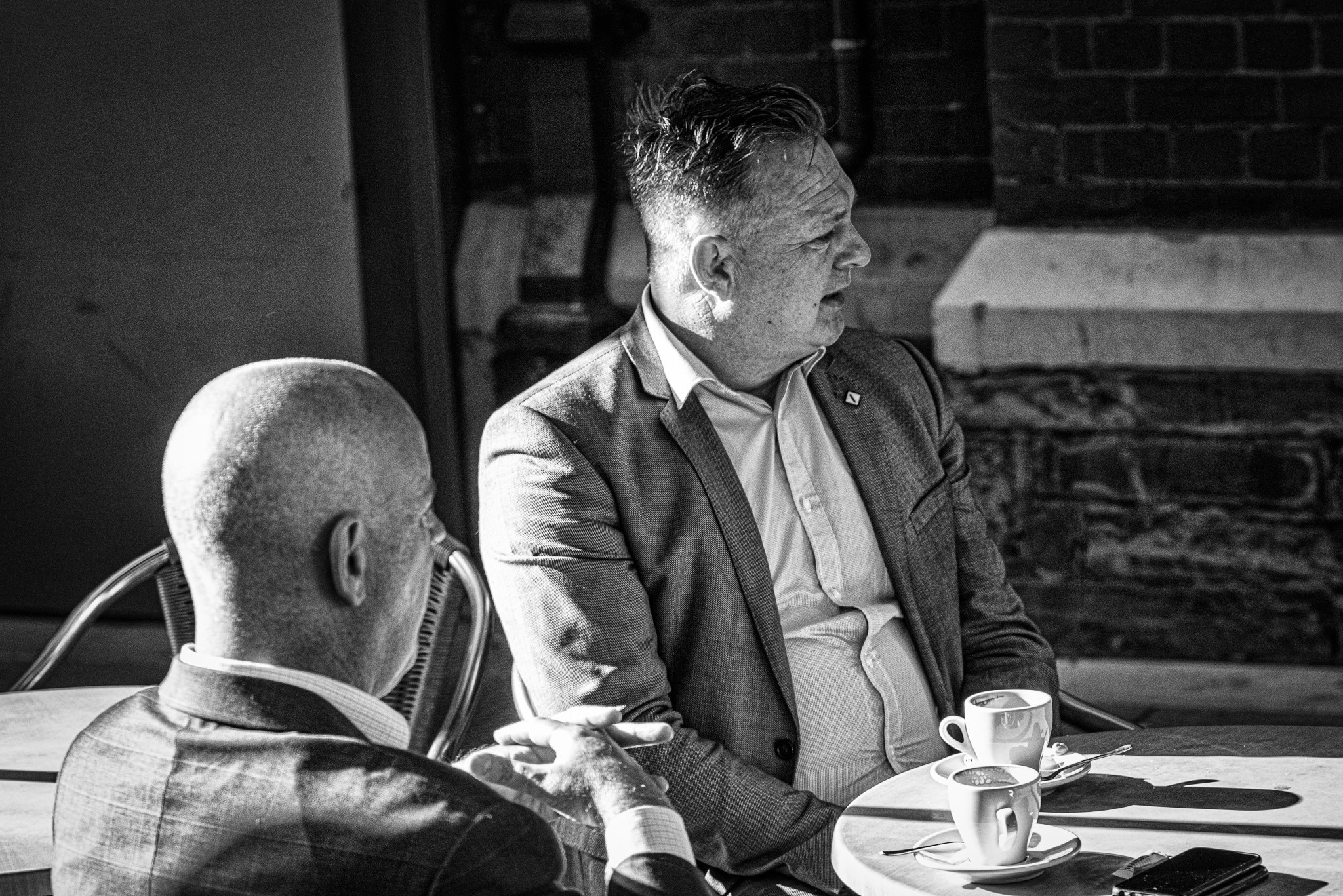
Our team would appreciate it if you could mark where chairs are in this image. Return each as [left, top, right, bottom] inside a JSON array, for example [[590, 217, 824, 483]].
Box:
[[8, 534, 496, 766]]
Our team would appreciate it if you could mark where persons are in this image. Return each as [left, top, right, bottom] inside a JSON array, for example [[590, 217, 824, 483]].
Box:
[[52, 359, 716, 895], [480, 68, 1060, 896]]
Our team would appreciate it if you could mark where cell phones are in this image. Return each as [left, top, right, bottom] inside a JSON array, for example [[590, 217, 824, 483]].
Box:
[[1112, 847, 1269, 896]]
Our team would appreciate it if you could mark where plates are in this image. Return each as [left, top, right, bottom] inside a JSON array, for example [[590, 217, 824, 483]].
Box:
[[929, 748, 1091, 798], [912, 823, 1082, 885]]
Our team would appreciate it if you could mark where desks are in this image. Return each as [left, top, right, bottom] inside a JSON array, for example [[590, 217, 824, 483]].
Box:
[[831, 725, 1343, 896], [0, 685, 153, 896]]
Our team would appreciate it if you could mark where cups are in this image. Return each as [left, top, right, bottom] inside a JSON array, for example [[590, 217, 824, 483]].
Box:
[[948, 762, 1042, 866], [938, 689, 1053, 775]]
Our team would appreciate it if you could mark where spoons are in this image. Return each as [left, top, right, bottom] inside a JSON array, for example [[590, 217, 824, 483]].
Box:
[[1038, 744, 1132, 781]]
